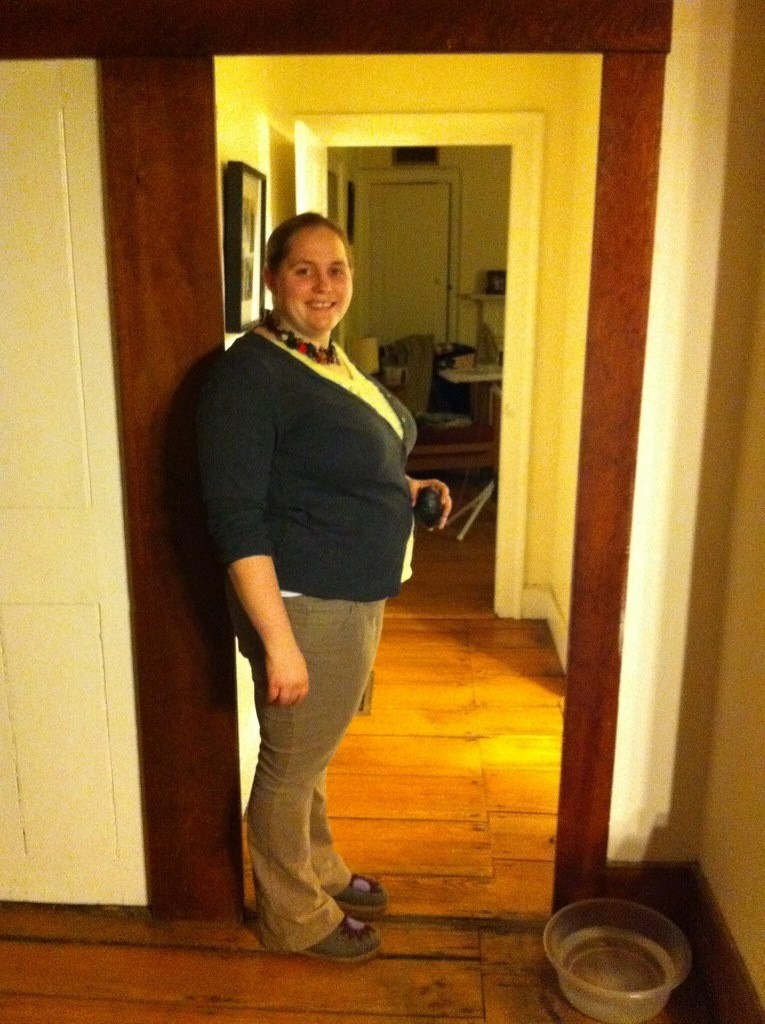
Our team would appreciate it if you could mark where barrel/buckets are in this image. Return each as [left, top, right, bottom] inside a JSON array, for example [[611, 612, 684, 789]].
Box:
[[429, 343, 477, 416]]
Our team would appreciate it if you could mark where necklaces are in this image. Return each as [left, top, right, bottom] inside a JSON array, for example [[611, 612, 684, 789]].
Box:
[[259, 308, 338, 363]]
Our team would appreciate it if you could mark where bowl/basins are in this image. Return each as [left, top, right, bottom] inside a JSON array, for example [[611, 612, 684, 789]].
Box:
[[543, 898, 693, 1024]]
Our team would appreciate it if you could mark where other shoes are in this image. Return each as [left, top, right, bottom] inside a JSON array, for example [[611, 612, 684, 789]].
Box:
[[332, 872, 387, 911], [294, 914, 381, 962]]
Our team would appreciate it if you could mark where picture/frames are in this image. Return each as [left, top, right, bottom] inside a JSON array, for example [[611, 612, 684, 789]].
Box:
[[224, 161, 266, 334]]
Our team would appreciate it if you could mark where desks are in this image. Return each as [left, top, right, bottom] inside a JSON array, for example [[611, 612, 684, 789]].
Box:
[[441, 367, 502, 424]]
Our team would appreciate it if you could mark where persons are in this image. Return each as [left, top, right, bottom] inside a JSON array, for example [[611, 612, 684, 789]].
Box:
[[196, 214, 452, 962]]
